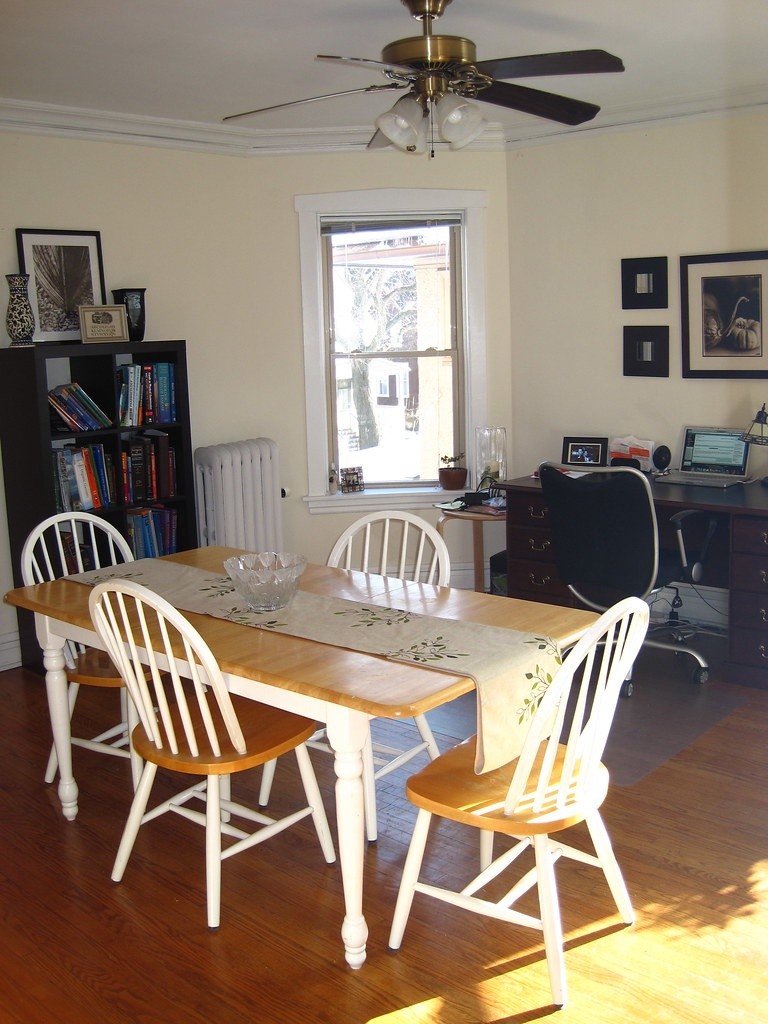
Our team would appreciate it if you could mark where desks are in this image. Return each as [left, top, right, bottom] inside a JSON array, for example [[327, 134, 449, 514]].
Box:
[[4, 543, 603, 971], [491, 472, 768, 690], [436, 509, 508, 593]]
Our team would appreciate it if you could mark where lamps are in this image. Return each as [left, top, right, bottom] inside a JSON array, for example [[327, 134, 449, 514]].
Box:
[[375, 72, 489, 157], [736, 401, 768, 447]]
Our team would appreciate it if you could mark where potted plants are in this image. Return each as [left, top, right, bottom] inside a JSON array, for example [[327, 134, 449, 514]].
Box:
[[438, 452, 468, 490]]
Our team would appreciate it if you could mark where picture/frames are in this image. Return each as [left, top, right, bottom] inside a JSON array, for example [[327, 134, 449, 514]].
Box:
[[79, 305, 130, 344], [15, 228, 108, 345], [341, 467, 364, 493], [562, 437, 609, 467], [679, 250, 768, 379]]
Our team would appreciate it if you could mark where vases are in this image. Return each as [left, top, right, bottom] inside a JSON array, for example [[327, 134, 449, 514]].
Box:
[[111, 288, 147, 342], [5, 273, 36, 347]]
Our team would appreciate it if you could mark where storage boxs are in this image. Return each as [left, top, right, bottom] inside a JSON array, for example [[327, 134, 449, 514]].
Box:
[[646, 581, 729, 629]]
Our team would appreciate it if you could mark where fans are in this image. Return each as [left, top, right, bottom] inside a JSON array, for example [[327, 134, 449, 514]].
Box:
[[223, 0, 626, 148]]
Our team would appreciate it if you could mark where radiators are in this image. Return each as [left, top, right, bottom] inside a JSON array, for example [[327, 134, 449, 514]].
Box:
[[194, 436, 285, 554]]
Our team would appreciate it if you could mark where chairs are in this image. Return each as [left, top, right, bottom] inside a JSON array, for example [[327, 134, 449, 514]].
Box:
[[538, 461, 719, 698], [388, 596, 648, 1004], [22, 512, 170, 793], [88, 578, 336, 930], [304, 510, 452, 842]]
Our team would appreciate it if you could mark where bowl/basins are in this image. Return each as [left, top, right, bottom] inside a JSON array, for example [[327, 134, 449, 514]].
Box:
[[223, 550, 307, 611]]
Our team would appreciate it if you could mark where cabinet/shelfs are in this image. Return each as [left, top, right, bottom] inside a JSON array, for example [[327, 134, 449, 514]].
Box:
[[0, 339, 197, 677]]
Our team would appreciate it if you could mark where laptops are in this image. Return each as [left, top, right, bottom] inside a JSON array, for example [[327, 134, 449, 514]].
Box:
[[655, 426, 751, 488]]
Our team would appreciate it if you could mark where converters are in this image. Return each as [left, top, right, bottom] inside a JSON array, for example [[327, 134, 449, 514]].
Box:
[[465, 492, 489, 505]]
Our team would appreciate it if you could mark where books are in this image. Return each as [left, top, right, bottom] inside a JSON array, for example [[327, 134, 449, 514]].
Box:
[[47, 383, 113, 432], [117, 362, 176, 427], [52, 443, 118, 514], [125, 506, 177, 561], [59, 531, 96, 575], [122, 436, 178, 505]]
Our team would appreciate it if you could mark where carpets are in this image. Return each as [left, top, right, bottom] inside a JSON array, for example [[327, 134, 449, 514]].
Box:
[[391, 670, 763, 784]]
